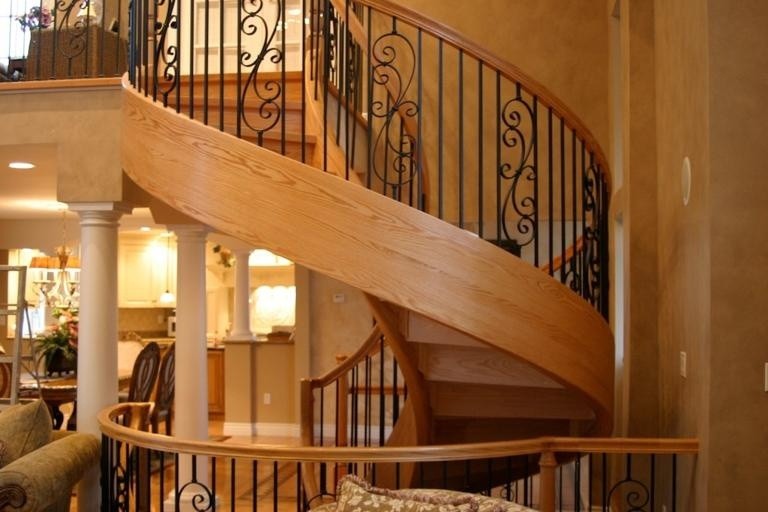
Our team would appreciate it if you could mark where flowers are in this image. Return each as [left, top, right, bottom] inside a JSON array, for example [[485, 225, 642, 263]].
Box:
[[27, 302, 79, 377]]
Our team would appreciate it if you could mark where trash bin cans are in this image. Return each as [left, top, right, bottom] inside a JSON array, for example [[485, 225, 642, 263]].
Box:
[[166, 311, 175, 338]]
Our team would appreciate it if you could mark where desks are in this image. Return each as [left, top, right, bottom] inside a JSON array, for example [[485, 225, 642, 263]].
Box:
[[19, 369, 132, 430]]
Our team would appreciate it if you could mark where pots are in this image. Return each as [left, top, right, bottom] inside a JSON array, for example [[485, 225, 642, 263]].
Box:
[[256, 332, 292, 340]]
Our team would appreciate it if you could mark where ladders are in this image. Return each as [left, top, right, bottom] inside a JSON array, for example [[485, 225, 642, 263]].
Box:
[[0, 265, 42, 405]]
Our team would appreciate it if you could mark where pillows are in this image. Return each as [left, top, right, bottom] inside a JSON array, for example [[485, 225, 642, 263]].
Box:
[[332, 473, 479, 512], [0, 397, 54, 469]]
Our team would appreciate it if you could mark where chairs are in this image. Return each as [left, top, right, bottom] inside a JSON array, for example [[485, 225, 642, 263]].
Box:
[[117, 342, 179, 465], [0, 409, 104, 512]]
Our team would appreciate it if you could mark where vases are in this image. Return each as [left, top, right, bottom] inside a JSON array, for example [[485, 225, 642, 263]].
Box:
[[45, 352, 76, 376]]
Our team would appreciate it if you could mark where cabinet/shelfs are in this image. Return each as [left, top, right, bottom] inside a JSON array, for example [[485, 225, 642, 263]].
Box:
[[205, 349, 224, 422], [118, 230, 177, 310]]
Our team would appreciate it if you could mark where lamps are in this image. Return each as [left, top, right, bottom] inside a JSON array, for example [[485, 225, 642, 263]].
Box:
[[160, 229, 176, 304], [31, 210, 81, 313]]
